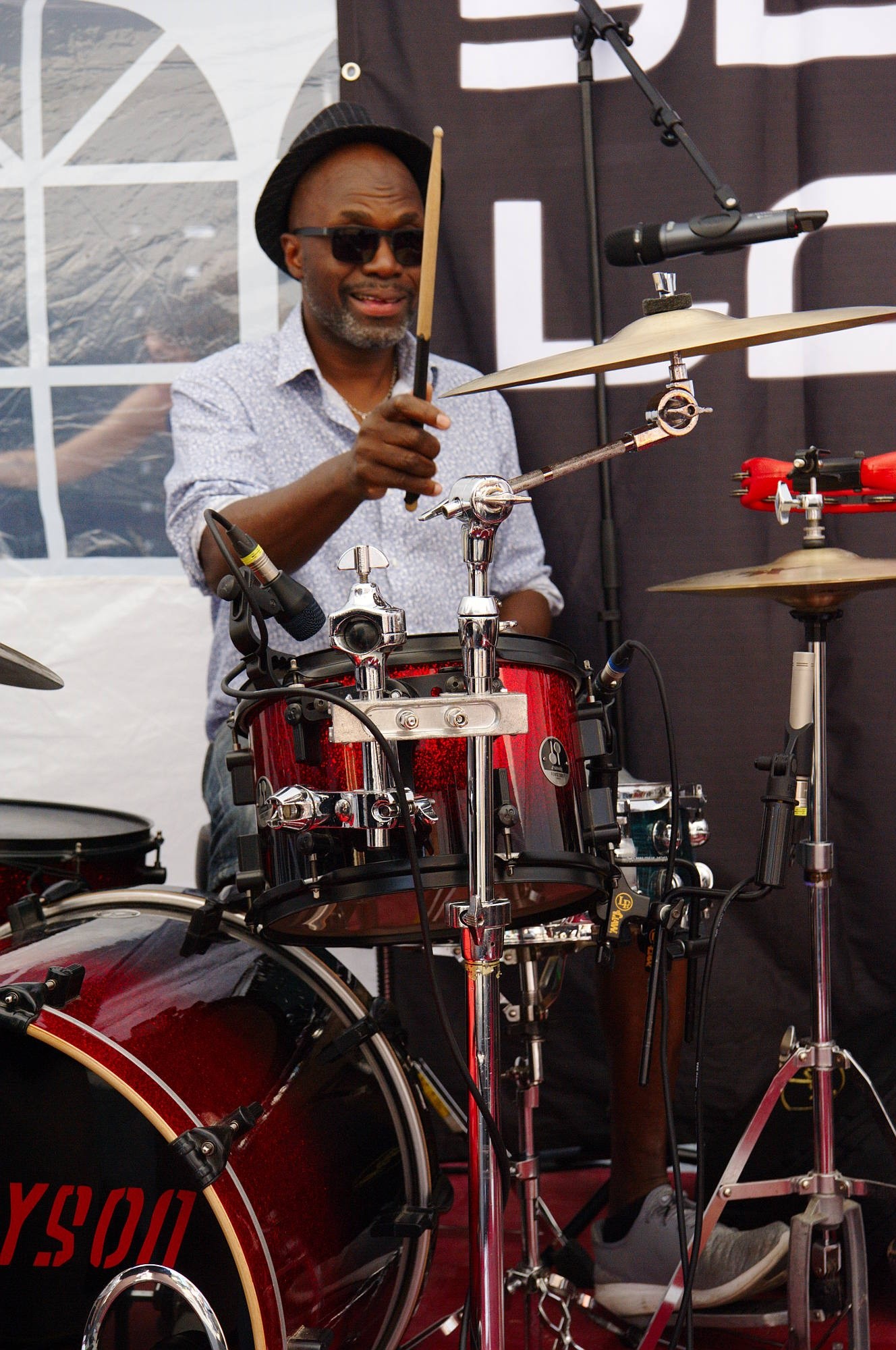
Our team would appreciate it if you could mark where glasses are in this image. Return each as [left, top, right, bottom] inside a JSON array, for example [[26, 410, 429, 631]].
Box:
[[290, 224, 424, 266]]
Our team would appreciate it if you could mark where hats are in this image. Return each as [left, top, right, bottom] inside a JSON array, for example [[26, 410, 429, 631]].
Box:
[[256, 101, 446, 280]]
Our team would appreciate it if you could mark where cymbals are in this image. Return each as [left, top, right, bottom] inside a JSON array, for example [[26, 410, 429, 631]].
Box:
[[0, 642, 65, 690], [644, 550, 896, 608], [437, 307, 896, 398]]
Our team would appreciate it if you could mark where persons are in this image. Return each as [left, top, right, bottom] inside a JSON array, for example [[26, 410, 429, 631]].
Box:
[[162, 105, 567, 904]]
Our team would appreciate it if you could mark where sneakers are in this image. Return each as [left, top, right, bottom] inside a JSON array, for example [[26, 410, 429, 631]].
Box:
[[589, 1183, 790, 1318]]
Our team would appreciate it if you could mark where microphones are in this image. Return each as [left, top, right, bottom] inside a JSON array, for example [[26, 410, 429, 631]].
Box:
[[788, 649, 816, 847], [578, 639, 637, 710], [227, 524, 326, 642], [603, 209, 829, 269]]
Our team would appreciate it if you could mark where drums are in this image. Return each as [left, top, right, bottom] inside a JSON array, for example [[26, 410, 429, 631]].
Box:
[[233, 632, 620, 947], [431, 784, 707, 955], [0, 884, 436, 1350], [0, 797, 154, 926]]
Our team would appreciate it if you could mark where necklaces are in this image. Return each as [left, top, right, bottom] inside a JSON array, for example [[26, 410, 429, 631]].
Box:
[[343, 363, 397, 420]]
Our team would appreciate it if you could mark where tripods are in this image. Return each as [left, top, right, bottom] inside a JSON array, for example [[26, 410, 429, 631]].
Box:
[[404, 604, 896, 1350]]
